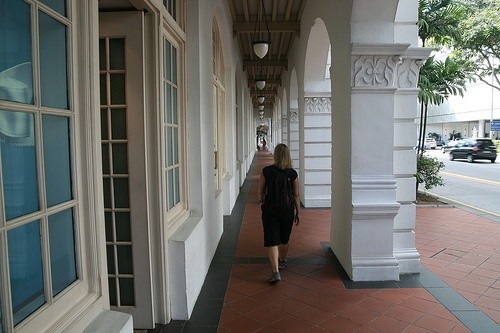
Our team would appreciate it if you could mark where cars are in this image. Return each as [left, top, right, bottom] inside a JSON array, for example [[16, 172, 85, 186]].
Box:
[[441, 141, 463, 154], [417, 138, 437, 149]]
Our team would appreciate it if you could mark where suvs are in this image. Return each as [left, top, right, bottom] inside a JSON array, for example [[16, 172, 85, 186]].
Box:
[[448, 138, 497, 163]]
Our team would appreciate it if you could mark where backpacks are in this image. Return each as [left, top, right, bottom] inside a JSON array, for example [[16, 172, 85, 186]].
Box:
[[267, 168, 294, 210]]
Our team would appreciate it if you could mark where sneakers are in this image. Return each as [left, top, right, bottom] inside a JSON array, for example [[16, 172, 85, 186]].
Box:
[[279, 260, 287, 268], [268, 272, 281, 283]]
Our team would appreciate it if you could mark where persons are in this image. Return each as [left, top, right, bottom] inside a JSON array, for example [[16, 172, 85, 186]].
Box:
[[258, 143, 300, 283], [263, 139, 266, 147]]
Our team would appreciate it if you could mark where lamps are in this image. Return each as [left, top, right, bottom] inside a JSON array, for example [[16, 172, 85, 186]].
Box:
[[251, 0, 272, 119]]
